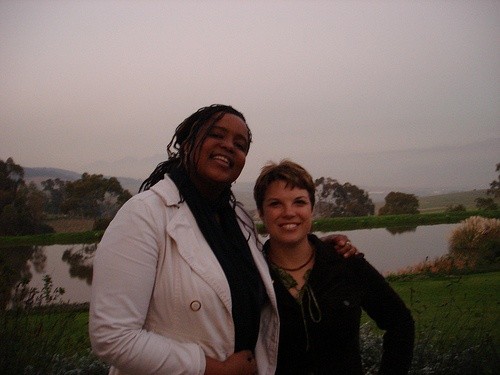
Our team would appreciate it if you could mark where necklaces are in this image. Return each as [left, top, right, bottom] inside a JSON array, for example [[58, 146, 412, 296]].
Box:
[[267, 249, 314, 271]]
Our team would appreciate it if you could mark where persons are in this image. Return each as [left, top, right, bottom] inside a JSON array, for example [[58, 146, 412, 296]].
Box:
[[253, 159, 416, 375], [89, 104, 358, 375]]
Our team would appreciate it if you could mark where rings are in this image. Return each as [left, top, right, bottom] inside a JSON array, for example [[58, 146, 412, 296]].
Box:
[[346, 240, 351, 248]]
